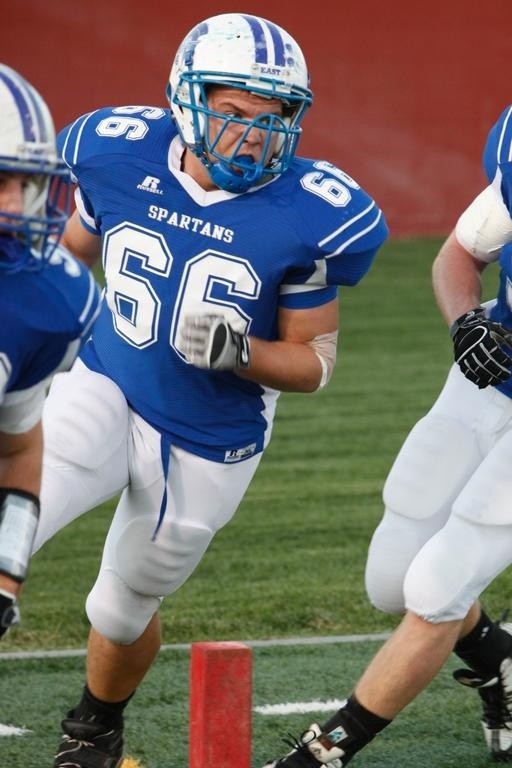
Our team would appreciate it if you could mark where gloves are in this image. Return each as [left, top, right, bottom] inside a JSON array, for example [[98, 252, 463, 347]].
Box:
[[0, 590, 19, 639], [177, 308, 249, 371], [450, 307, 512, 389]]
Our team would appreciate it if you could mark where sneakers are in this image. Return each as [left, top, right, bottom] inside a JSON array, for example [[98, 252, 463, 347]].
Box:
[[452, 622, 512, 758], [52, 709, 122, 768], [263, 723, 347, 768]]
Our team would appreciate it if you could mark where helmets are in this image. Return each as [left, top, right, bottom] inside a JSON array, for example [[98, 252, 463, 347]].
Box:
[[166, 12, 313, 148], [0, 63, 71, 243]]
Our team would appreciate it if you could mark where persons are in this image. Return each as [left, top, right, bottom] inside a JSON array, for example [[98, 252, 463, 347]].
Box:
[[0, 64, 101, 642], [263, 104, 512, 768], [30, 13, 389, 768]]
